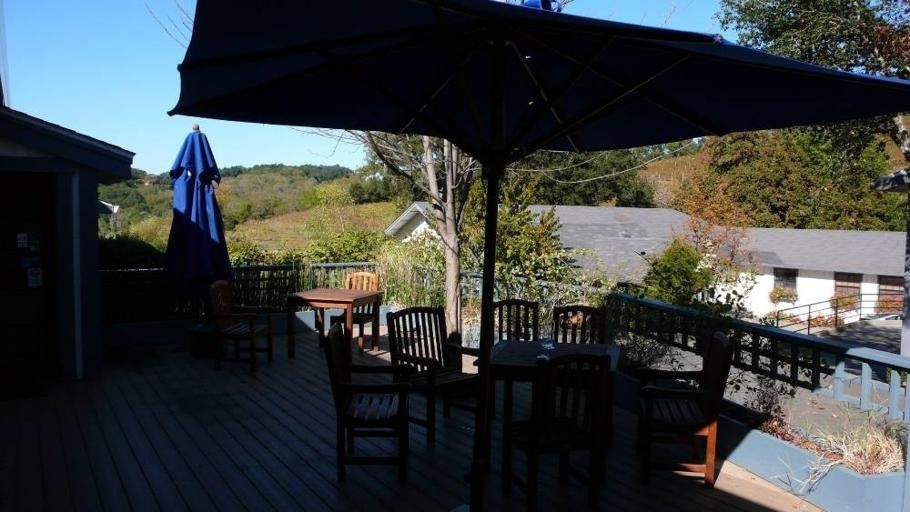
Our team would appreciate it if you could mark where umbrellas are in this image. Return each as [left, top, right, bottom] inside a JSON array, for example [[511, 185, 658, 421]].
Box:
[[163, 123, 235, 360], [159, 0, 910, 511]]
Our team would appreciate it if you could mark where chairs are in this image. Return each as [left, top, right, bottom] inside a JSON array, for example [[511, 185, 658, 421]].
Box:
[[211, 280, 273, 374], [331, 273, 381, 354], [387, 305, 494, 443], [638, 333, 735, 487], [489, 298, 541, 346], [553, 306, 607, 344], [320, 323, 416, 482], [500, 352, 614, 512]]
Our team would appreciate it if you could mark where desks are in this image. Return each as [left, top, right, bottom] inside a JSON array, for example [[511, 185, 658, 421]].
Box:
[[474, 341, 625, 465], [287, 288, 383, 367]]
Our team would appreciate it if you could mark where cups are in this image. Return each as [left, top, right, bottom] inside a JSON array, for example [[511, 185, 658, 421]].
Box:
[[537, 317, 556, 350]]
